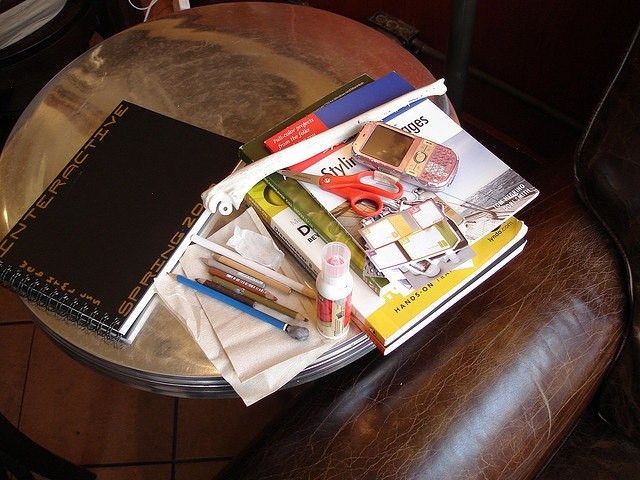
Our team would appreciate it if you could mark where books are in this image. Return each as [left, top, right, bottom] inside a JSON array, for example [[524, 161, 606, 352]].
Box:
[[263, 71, 540, 297], [237, 72, 398, 296], [245, 161, 529, 346], [263, 220, 527, 356], [0, 100, 244, 345]]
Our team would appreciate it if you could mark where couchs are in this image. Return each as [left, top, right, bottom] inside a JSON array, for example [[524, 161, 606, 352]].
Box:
[[210, 24, 640, 479]]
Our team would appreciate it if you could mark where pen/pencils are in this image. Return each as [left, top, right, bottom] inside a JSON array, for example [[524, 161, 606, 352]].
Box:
[[212, 253, 294, 296], [194, 278, 257, 308], [166, 272, 310, 342], [208, 266, 278, 303], [190, 234, 317, 300], [199, 257, 266, 288], [211, 275, 309, 323]]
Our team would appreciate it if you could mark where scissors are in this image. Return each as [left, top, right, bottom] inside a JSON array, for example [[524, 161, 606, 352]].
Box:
[[278, 170, 403, 217]]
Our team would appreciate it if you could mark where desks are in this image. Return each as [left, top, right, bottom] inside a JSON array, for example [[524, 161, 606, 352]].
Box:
[[0, 1, 461, 399]]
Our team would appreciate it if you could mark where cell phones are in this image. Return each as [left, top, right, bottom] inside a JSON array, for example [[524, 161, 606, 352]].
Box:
[[351, 120, 459, 191]]
